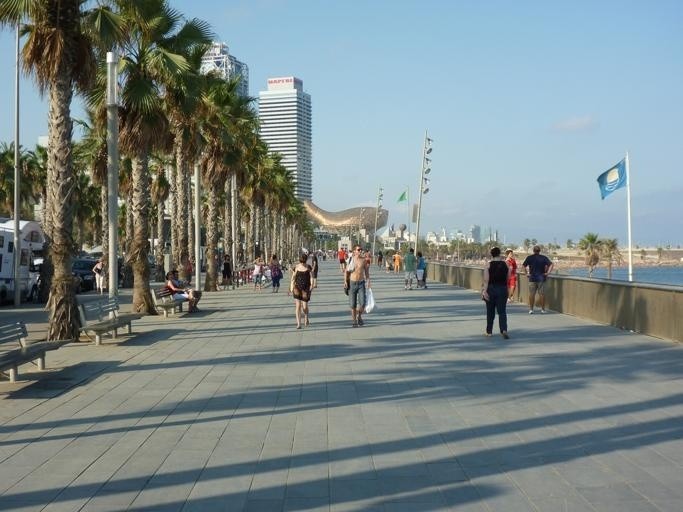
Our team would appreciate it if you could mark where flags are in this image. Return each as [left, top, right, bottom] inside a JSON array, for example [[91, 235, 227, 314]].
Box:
[[395, 188, 407, 204], [596, 157, 626, 199]]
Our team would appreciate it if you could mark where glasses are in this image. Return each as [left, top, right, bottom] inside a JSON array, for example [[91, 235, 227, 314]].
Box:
[[355, 248, 362, 251]]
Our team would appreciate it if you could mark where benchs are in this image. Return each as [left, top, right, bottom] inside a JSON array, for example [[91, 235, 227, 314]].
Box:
[[1, 316, 74, 383], [150, 285, 187, 318], [76, 292, 146, 345]]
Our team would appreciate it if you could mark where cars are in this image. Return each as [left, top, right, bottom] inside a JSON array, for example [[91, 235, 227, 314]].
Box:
[[33, 251, 157, 296]]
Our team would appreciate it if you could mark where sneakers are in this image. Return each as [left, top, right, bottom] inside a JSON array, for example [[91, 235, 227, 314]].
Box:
[[528, 310, 534, 314], [352, 321, 358, 328], [484, 331, 510, 340], [356, 315, 363, 325], [540, 309, 546, 314]]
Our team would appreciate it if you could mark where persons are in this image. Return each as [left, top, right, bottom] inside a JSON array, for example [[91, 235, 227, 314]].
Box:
[[415, 251, 425, 288], [91, 257, 105, 293], [173, 270, 201, 313], [343, 244, 370, 328], [403, 247, 416, 290], [308, 252, 318, 290], [480, 246, 510, 340], [222, 255, 232, 290], [503, 249, 517, 303], [375, 249, 402, 273], [268, 254, 281, 293], [252, 256, 263, 291], [165, 271, 197, 313], [289, 253, 314, 330], [278, 256, 292, 274], [521, 244, 553, 315], [318, 247, 370, 273], [184, 261, 192, 286]]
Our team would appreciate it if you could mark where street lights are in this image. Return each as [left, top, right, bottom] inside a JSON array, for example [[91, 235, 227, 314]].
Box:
[[356, 208, 369, 245], [413, 127, 433, 259], [371, 184, 386, 257]]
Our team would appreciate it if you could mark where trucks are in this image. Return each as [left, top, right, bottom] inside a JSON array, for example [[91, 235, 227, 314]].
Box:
[[0, 215, 47, 303]]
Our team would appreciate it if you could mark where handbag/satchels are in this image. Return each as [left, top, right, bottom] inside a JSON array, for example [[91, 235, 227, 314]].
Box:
[[343, 279, 350, 295], [364, 288, 379, 314]]
